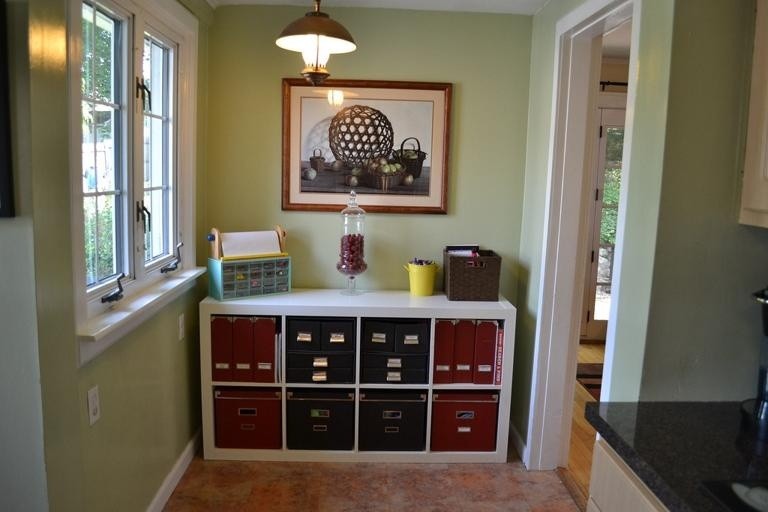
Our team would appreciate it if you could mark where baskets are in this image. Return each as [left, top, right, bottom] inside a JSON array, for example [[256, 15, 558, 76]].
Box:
[[442, 250, 501, 302], [392, 137, 426, 178], [365, 146, 407, 190], [309, 148, 325, 173]]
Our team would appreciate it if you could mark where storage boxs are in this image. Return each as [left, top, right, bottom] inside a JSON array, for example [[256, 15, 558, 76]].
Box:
[[208, 315, 499, 452]]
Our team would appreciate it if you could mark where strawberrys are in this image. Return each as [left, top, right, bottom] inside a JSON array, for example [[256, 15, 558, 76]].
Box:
[[337, 234, 367, 274]]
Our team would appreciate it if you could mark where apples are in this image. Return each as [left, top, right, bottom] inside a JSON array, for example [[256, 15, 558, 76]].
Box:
[[402, 174, 414, 186], [304, 168, 317, 181], [367, 157, 402, 174], [351, 167, 363, 176], [330, 160, 344, 172], [344, 174, 358, 187]]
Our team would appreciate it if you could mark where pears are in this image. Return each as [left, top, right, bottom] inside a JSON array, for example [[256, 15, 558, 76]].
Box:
[[396, 148, 419, 159]]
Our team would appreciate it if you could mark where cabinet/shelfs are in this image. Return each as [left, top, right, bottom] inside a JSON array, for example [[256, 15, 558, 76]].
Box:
[[199, 287, 518, 465], [737, 0, 768, 228], [586, 433, 669, 512]]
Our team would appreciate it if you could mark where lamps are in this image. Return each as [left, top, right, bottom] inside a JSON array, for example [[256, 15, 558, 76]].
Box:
[[275, 0, 357, 87]]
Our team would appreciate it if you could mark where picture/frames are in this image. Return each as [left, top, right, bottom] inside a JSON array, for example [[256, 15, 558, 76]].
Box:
[[283, 78, 453, 214]]
